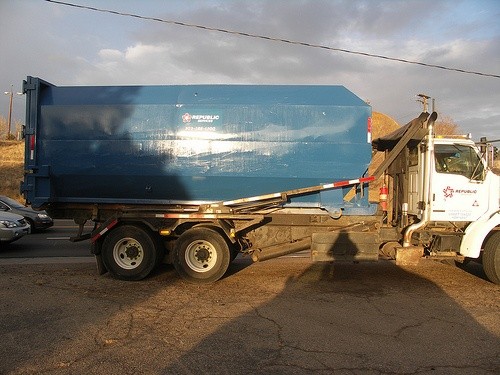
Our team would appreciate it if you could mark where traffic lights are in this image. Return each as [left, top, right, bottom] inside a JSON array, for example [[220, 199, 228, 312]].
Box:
[[492, 146, 498, 159]]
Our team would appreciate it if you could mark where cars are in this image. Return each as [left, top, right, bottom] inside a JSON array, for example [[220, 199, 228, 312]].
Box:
[[0, 196, 53, 231], [0, 210, 30, 243]]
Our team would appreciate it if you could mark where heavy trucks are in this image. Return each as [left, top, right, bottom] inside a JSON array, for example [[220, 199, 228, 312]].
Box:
[[19, 76, 499, 285]]
